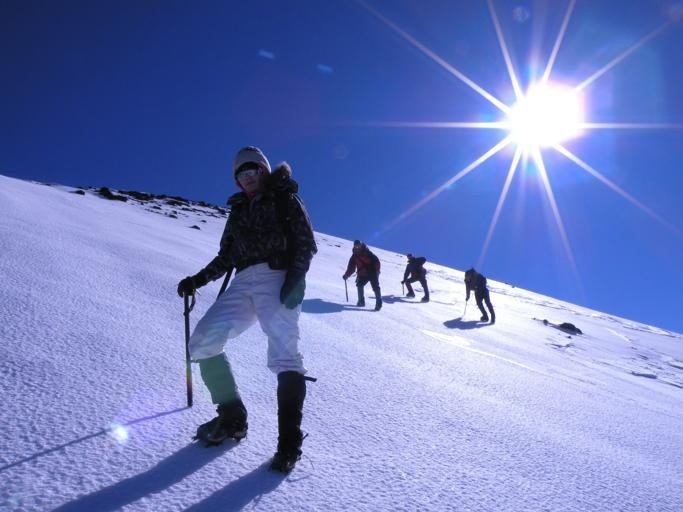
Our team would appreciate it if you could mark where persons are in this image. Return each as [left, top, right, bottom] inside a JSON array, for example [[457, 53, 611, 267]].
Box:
[[176, 145, 319, 475], [341, 239, 383, 311], [464, 267, 497, 324], [400, 252, 431, 302]]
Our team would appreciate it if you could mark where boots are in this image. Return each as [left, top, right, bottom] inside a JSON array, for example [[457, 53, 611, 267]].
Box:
[[195, 352, 248, 443], [272, 371, 307, 472]]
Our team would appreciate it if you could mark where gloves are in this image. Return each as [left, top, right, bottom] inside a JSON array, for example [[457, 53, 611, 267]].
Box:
[[178, 276, 196, 297], [280, 271, 306, 309]]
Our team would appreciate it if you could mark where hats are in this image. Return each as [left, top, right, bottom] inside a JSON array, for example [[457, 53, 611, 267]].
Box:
[[234, 145, 271, 175]]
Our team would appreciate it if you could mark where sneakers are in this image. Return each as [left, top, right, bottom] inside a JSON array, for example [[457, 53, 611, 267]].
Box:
[[407, 291, 430, 301], [479, 313, 497, 323], [356, 298, 382, 311]]
